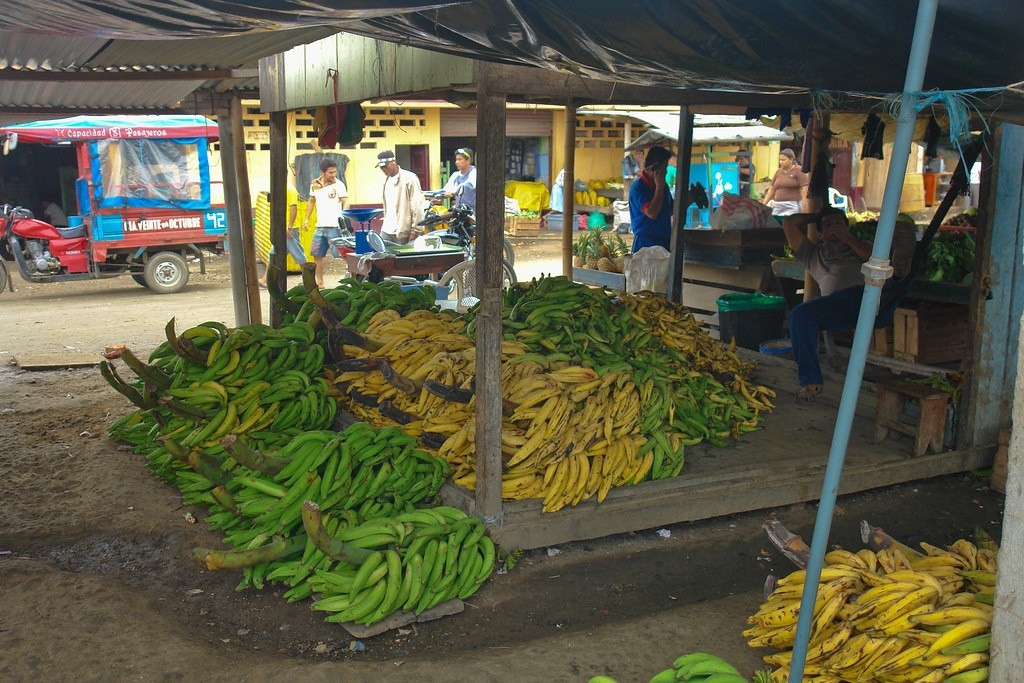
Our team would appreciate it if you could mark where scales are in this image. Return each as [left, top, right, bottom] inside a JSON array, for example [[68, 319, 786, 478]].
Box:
[[342, 207, 386, 254]]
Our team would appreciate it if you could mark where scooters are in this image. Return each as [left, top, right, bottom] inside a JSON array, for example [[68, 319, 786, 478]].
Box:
[[329, 187, 515, 281]]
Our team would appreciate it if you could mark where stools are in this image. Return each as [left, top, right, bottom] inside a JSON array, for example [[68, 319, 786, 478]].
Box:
[[873, 378, 952, 458]]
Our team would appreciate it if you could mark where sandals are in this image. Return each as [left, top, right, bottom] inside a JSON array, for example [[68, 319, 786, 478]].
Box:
[[794, 384, 824, 404], [892, 221, 917, 277]]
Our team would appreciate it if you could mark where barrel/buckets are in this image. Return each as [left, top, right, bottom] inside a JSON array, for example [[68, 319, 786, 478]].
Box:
[[759, 339, 794, 360], [68, 216, 82, 227], [716, 291, 786, 352]]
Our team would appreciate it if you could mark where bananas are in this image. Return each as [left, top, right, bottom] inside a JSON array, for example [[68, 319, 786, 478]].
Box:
[[585, 652, 750, 683], [105, 271, 776, 625], [743, 525, 1005, 683]]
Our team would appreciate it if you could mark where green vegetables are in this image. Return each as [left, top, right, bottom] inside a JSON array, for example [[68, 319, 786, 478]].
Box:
[[770, 243, 798, 261], [916, 231, 975, 282], [849, 218, 878, 245]]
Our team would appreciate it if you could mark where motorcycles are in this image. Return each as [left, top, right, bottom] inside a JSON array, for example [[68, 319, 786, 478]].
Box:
[[0, 112, 227, 297]]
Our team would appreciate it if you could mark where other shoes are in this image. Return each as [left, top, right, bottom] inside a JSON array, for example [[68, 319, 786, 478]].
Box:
[[258, 280, 267, 288]]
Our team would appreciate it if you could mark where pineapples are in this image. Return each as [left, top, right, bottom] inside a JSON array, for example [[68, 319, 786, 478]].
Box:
[[571, 226, 632, 275]]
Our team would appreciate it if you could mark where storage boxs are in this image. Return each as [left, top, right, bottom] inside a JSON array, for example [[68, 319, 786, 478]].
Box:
[[509, 216, 540, 237], [548, 214, 578, 231]]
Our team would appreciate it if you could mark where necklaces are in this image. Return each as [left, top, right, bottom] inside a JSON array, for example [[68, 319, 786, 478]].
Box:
[[784, 165, 795, 174]]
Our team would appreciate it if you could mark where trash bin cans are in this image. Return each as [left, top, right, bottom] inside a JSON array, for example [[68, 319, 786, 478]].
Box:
[[717, 293, 786, 352]]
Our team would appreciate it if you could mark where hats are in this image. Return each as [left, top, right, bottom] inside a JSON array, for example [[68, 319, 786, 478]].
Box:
[[455, 147, 473, 164], [375, 150, 395, 168]]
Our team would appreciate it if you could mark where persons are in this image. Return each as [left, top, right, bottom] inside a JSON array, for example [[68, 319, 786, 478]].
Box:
[[542, 166, 564, 221], [441, 147, 477, 219], [36, 197, 68, 228], [304, 156, 350, 289], [625, 147, 701, 254], [258, 169, 309, 288], [761, 148, 809, 217], [0, 237, 29, 261], [783, 208, 913, 403], [621, 149, 642, 202], [374, 150, 425, 246], [735, 150, 755, 197]]
